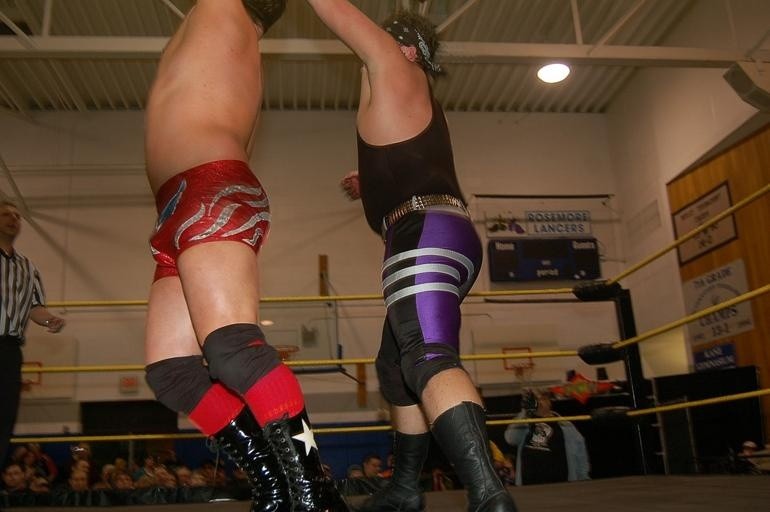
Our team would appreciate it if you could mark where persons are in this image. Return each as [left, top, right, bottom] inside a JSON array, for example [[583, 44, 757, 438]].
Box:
[[308, 0, 519, 512], [2, 199, 67, 489], [142, 1, 350, 512], [1, 391, 594, 512]]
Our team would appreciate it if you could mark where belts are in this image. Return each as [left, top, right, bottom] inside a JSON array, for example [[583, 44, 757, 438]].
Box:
[[380, 192, 470, 243]]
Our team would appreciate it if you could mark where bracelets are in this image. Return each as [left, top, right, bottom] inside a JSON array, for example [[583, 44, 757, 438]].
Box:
[[44, 316, 55, 327]]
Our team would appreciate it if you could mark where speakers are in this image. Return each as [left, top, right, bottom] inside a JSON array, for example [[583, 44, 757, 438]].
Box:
[[316, 252, 331, 294]]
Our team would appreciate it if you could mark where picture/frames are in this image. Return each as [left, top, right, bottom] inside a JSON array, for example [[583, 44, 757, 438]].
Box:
[[671, 179, 738, 267]]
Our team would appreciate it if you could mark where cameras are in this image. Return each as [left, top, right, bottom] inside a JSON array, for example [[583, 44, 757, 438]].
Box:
[[519, 388, 543, 415]]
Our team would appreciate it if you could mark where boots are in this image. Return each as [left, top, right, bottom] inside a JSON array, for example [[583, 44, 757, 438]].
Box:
[[432, 401, 515, 512], [360, 428, 431, 512], [206, 403, 350, 512]]
